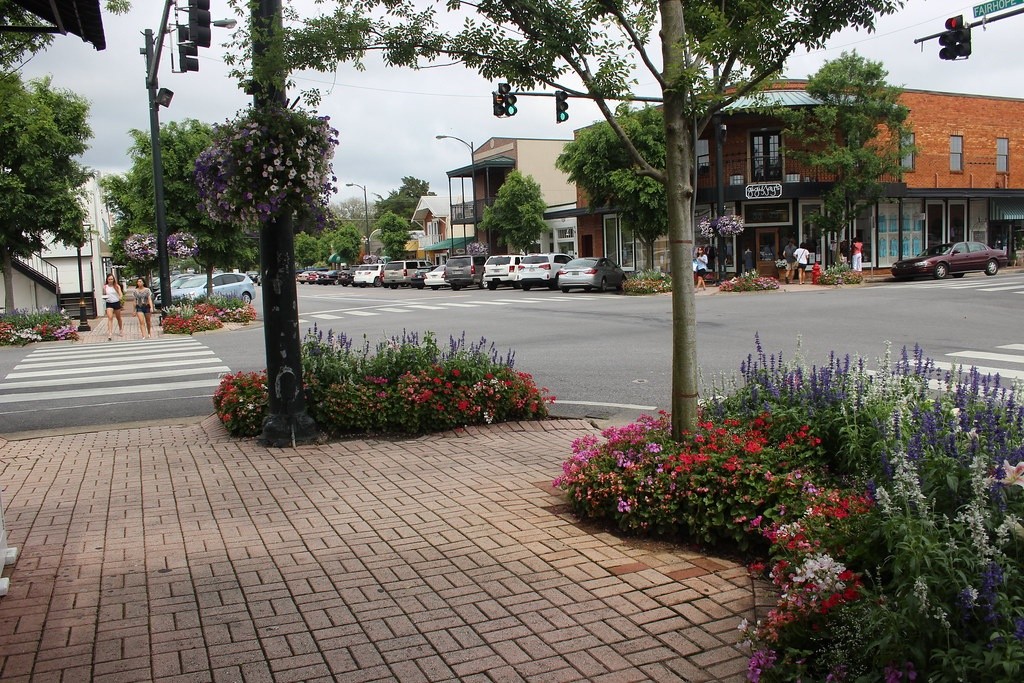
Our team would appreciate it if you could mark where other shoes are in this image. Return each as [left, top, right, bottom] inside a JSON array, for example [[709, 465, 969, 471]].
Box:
[[785, 276, 788, 283]]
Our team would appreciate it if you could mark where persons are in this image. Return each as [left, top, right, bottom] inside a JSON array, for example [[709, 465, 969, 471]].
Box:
[[695, 247, 709, 292], [850, 237, 864, 272], [793, 241, 809, 285], [132, 277, 153, 339], [782, 238, 798, 283], [101, 273, 124, 340]]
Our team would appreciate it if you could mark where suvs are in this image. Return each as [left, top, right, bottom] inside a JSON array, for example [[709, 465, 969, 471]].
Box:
[[482, 254, 526, 290], [518, 253, 574, 291]]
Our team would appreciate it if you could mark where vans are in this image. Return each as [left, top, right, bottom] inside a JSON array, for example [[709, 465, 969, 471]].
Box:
[[443, 255, 491, 290]]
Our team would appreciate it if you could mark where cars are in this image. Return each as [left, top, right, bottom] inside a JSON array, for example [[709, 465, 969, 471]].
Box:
[[558, 257, 628, 293], [892, 241, 1008, 280], [424, 264, 451, 290], [128, 272, 256, 307], [247, 258, 442, 291]]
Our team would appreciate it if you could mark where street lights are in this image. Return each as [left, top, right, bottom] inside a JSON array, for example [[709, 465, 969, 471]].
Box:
[[346, 182, 371, 264], [435, 134, 478, 244]]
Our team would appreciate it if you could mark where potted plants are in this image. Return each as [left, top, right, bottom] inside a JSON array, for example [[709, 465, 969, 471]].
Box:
[[783, 172, 801, 183], [730, 174, 744, 185]]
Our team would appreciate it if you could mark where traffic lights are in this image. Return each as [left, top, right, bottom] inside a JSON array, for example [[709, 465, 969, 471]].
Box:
[[498, 83, 511, 94], [938, 32, 957, 60], [187, 0, 212, 48], [555, 90, 570, 124], [493, 94, 505, 116], [177, 24, 199, 74], [505, 94, 518, 117], [954, 28, 971, 56], [944, 14, 963, 31], [157, 87, 174, 107]]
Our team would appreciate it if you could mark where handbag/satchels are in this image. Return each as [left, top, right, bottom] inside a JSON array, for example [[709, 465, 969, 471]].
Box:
[[693, 262, 698, 271], [793, 261, 798, 268]]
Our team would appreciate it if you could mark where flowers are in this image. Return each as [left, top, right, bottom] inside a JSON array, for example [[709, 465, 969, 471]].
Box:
[[466, 242, 489, 255], [124, 234, 157, 262], [364, 255, 377, 264], [194, 107, 340, 238], [696, 216, 716, 238], [166, 232, 199, 260], [717, 215, 744, 236]]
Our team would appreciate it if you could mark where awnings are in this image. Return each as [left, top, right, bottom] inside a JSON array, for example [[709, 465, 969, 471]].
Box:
[[989, 197, 1024, 220]]
